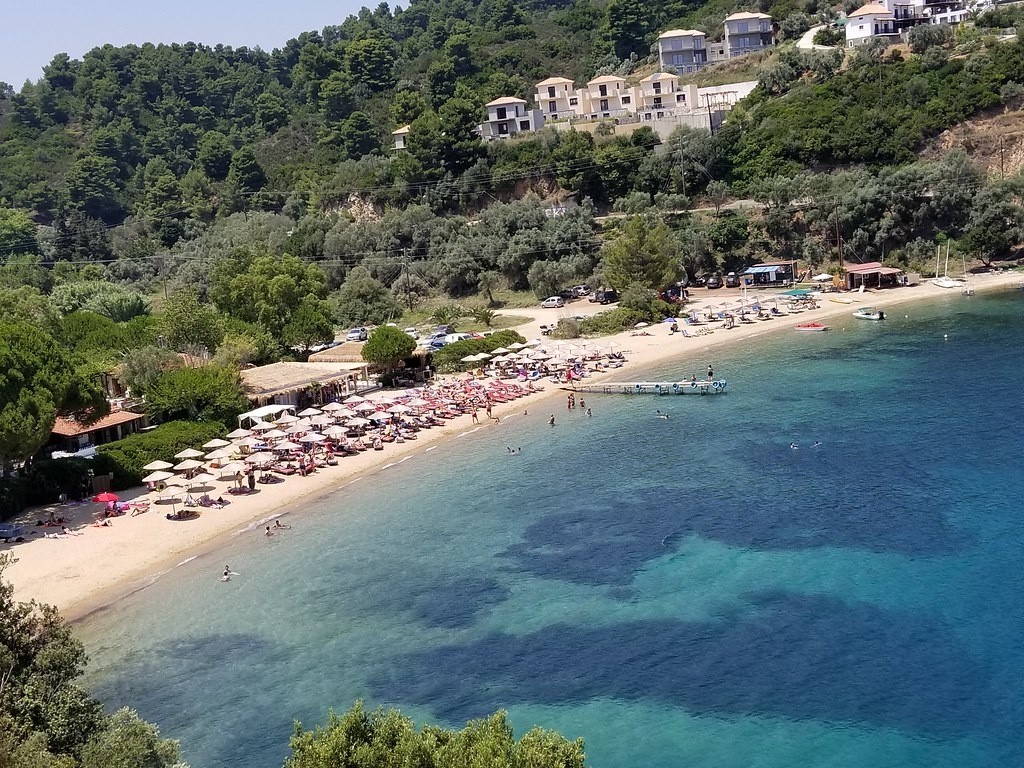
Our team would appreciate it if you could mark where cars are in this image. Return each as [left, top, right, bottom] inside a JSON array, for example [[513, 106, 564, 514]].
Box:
[[559, 284, 619, 305], [541, 296, 564, 309], [402, 323, 487, 351], [696, 270, 740, 289], [345, 327, 368, 341]]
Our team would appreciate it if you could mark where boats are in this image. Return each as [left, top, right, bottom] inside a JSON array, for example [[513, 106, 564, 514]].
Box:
[[795, 321, 830, 332], [854, 305, 888, 322]]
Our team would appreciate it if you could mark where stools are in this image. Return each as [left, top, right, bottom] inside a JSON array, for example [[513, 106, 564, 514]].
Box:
[[229, 377, 546, 474]]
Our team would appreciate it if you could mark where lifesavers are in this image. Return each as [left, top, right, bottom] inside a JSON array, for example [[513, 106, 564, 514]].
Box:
[[719, 381, 727, 388], [655, 384, 661, 390], [635, 384, 641, 389], [690, 382, 698, 389], [672, 383, 679, 390], [712, 381, 719, 389]]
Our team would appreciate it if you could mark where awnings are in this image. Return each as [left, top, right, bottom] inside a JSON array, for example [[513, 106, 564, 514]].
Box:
[[237, 404, 297, 430], [775, 289, 816, 297], [850, 267, 902, 287], [744, 266, 780, 273]]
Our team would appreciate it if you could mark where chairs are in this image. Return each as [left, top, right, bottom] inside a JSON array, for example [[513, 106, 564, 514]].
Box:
[[665, 288, 823, 337], [480, 351, 627, 377]]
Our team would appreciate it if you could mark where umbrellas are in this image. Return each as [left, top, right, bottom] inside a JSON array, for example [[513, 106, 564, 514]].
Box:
[[92, 491, 119, 511], [811, 272, 834, 283], [141, 394, 431, 514], [461, 339, 621, 374], [635, 321, 648, 332], [689, 294, 799, 318], [663, 317, 677, 327]]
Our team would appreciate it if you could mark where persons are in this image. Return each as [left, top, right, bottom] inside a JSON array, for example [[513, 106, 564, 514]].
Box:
[[265, 520, 287, 535], [43, 525, 85, 539], [96, 517, 113, 527], [470, 406, 479, 424], [707, 365, 714, 382], [222, 566, 231, 579], [548, 414, 554, 424], [690, 374, 696, 381], [486, 401, 492, 419], [568, 392, 592, 416]]
